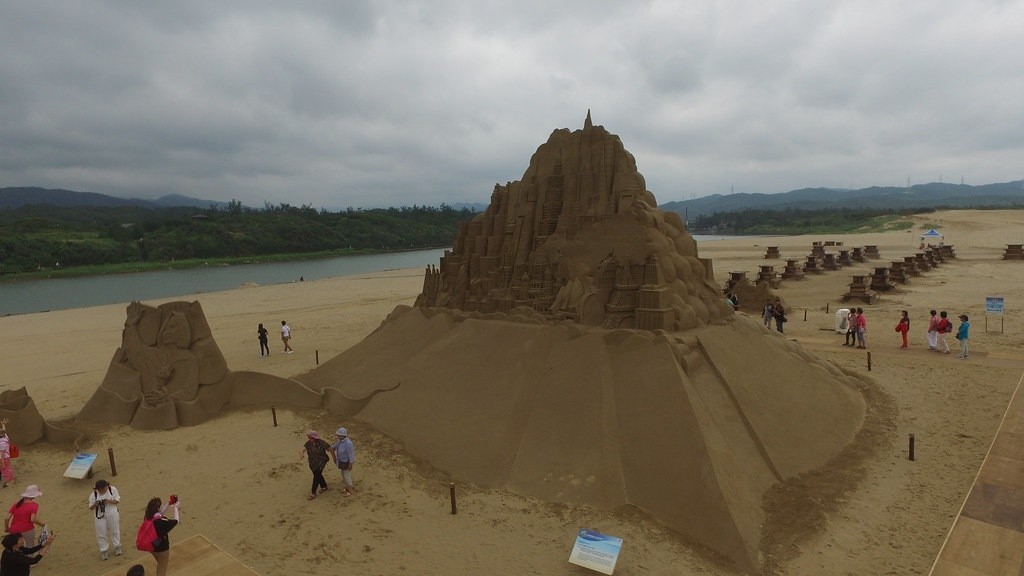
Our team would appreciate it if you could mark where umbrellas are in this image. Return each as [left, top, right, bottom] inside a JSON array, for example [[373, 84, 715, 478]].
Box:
[[919, 228, 942, 238]]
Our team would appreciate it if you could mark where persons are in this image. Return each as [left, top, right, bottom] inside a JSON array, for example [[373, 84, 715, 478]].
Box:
[[725, 292, 739, 310], [0, 418, 15, 487], [126, 564, 145, 576], [280, 321, 294, 354], [143, 496, 180, 576], [89, 479, 121, 560], [955, 314, 970, 359], [761, 298, 785, 333], [842, 307, 867, 348], [927, 310, 951, 354], [299, 431, 337, 500], [4, 485, 47, 566], [258, 323, 270, 357], [899, 310, 910, 349], [327, 428, 354, 497], [0, 529, 56, 576]]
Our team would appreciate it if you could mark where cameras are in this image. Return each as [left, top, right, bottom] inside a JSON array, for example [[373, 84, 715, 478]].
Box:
[[96, 499, 107, 513]]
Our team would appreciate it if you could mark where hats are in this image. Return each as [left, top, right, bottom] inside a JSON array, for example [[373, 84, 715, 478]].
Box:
[[336, 428, 347, 436], [307, 431, 319, 439], [93, 479, 109, 489], [22, 485, 42, 498], [958, 315, 968, 319]]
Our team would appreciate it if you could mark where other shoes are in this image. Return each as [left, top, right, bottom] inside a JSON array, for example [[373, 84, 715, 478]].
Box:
[[927, 346, 937, 351], [956, 355, 968, 360], [281, 349, 293, 354], [342, 487, 350, 496], [308, 494, 316, 500], [856, 346, 866, 350], [4, 480, 14, 487], [936, 350, 950, 354], [101, 552, 109, 560], [842, 343, 854, 347], [115, 548, 121, 555], [319, 487, 328, 493]]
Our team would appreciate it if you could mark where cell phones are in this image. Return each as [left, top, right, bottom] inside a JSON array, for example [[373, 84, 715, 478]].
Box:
[[174, 495, 178, 503]]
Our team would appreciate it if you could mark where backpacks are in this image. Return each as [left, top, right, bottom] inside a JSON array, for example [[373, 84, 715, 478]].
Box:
[[939, 318, 953, 333], [10, 444, 18, 457], [136, 521, 158, 551]]
